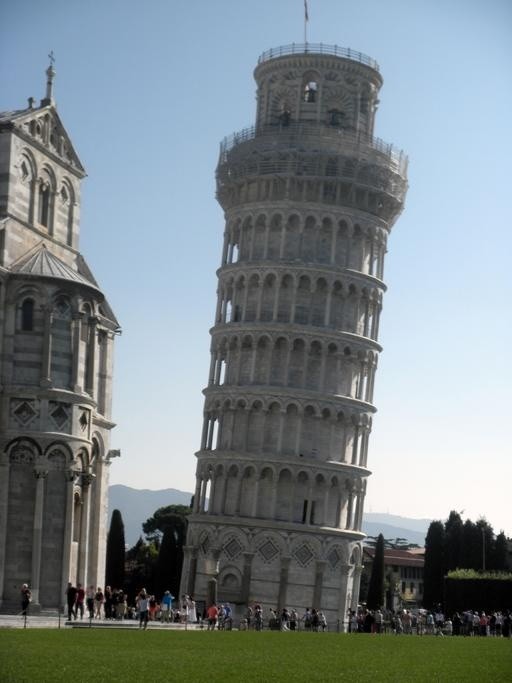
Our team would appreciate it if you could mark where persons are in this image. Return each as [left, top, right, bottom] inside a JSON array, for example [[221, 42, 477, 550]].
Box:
[[207, 602, 232, 630], [22, 583, 33, 615], [350, 608, 512, 637], [247, 605, 327, 632], [66, 583, 196, 629]]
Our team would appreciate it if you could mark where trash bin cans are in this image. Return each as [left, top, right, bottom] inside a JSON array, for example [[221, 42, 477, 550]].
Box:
[[239, 619, 247, 631]]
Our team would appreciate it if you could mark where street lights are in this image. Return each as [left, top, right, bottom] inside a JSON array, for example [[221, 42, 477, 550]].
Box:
[[479, 525, 487, 568]]
